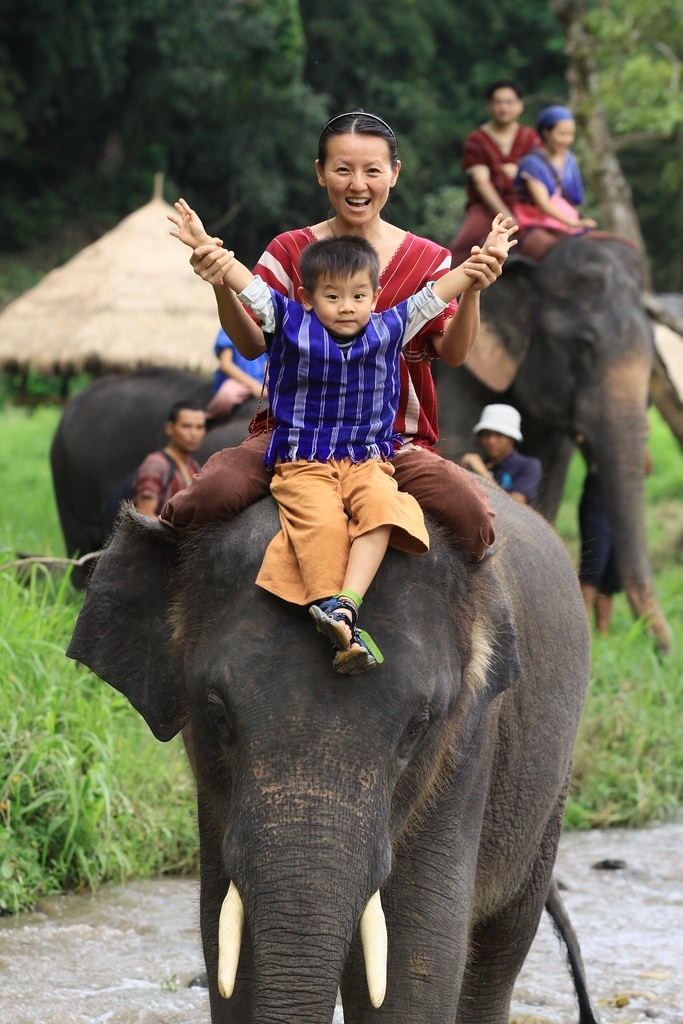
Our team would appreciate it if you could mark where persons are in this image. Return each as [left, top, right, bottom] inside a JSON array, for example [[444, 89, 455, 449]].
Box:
[[448, 79, 639, 304], [132, 400, 206, 519], [463, 405, 542, 504], [571, 428, 652, 634], [159, 107, 519, 674], [203, 328, 269, 426]]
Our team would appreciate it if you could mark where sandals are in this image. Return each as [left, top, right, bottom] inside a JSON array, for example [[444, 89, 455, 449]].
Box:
[[309, 589, 363, 650], [332, 628, 384, 676]]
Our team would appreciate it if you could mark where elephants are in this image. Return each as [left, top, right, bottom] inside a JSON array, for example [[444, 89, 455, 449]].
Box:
[[48, 369, 600, 1024], [429, 233, 682, 655]]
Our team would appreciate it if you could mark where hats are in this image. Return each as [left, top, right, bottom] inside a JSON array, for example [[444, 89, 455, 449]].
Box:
[[472, 404, 523, 442]]
